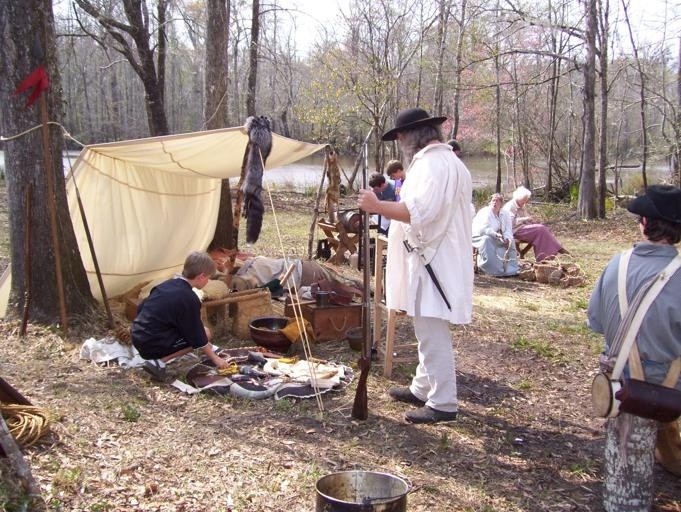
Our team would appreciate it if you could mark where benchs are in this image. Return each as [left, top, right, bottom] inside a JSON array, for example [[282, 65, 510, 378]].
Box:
[[473, 239, 532, 272]]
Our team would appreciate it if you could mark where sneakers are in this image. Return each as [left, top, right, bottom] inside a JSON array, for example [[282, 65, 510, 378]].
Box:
[[405, 404, 457, 425], [389, 386, 427, 406]]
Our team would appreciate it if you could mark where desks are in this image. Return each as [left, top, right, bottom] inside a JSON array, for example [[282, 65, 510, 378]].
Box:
[[125, 287, 273, 337], [318, 218, 359, 265]]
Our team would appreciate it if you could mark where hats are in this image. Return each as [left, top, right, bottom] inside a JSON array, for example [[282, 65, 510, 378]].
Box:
[[627, 185, 681, 225], [382, 108, 448, 141]]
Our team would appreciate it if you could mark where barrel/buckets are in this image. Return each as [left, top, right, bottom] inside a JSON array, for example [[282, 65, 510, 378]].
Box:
[[315, 471, 412, 512]]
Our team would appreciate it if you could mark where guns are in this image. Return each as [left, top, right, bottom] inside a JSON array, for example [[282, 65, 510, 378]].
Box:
[[351, 143, 371, 419]]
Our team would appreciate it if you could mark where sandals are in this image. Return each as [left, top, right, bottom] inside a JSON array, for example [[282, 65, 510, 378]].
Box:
[[141, 359, 176, 383]]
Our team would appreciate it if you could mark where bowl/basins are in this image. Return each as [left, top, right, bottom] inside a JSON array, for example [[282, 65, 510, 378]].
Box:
[[346, 327, 373, 350], [248, 317, 293, 352]]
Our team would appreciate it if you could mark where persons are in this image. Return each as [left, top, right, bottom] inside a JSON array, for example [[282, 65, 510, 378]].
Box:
[[366, 170, 395, 231], [128, 249, 229, 385], [582, 182, 680, 510], [471, 190, 520, 279], [446, 139, 461, 158], [500, 186, 573, 261], [355, 106, 478, 424], [210, 256, 242, 292], [385, 159, 407, 202]]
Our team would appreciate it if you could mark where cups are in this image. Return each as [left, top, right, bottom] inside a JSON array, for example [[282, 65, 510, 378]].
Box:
[[316, 290, 336, 307]]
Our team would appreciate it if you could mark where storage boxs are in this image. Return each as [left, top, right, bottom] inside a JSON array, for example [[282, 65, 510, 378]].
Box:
[[284, 294, 362, 343]]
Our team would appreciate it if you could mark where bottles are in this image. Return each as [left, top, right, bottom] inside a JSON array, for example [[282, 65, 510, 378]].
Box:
[[350, 250, 359, 269], [317, 240, 331, 259]]
